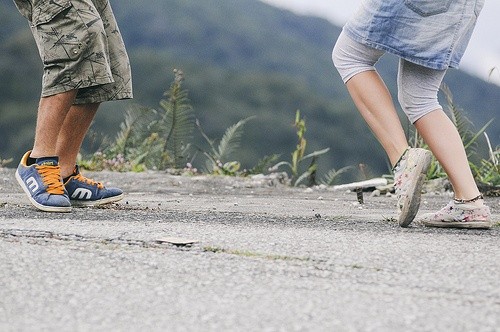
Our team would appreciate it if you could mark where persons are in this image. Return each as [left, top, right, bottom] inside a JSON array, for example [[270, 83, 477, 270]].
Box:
[[330, 0, 494, 229], [12, 0, 134, 213]]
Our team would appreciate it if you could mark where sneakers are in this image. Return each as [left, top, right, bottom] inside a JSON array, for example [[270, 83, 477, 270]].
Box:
[[420, 201, 490, 228], [393, 147, 432, 227], [15, 151, 71, 212], [63, 165, 125, 205]]
